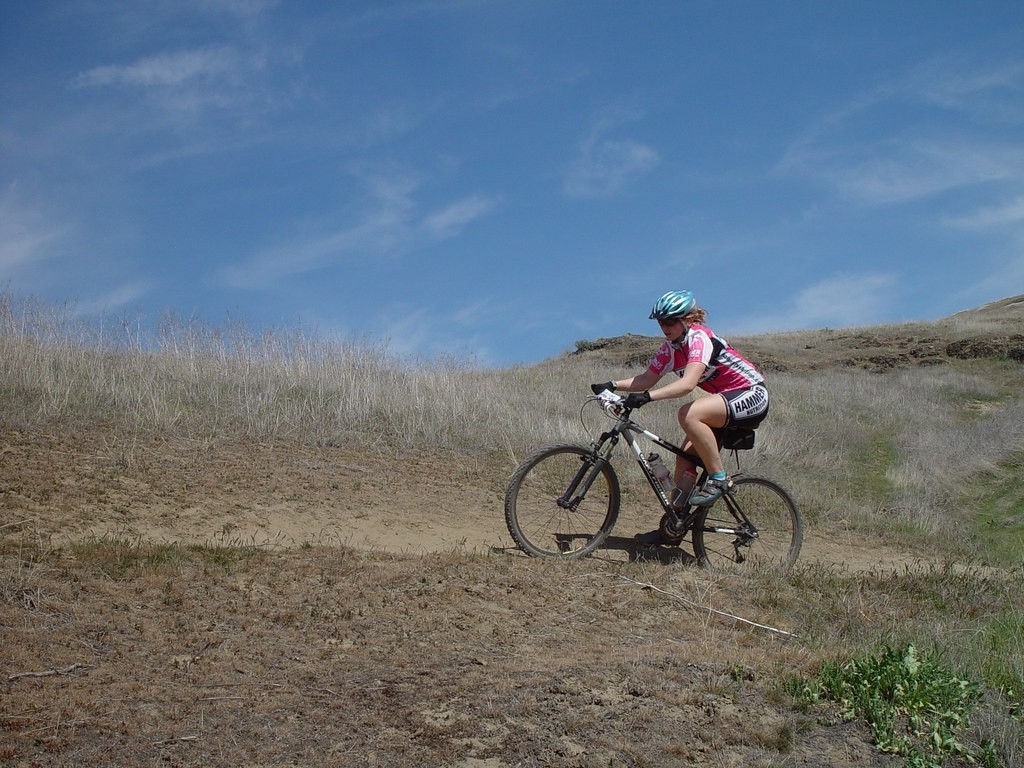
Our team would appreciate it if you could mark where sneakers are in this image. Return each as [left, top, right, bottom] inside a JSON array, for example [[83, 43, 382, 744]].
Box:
[[689, 474, 735, 506], [635, 530, 681, 547]]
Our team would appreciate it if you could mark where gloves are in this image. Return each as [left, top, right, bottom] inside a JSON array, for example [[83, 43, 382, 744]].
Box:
[[590, 381, 617, 395], [626, 391, 651, 408]]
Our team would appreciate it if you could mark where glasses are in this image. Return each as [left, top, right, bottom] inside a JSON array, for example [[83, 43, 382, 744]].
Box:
[[658, 317, 682, 327]]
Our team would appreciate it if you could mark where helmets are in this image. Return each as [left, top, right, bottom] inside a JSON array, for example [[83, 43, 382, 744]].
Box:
[[649, 290, 697, 320]]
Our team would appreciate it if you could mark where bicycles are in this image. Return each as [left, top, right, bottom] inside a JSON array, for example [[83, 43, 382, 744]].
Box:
[[502, 384, 804, 586]]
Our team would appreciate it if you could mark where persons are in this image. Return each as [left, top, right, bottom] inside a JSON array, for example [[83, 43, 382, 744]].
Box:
[[591, 291, 769, 548]]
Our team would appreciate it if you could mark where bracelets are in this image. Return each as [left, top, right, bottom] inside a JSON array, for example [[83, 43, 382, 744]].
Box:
[[611, 380, 617, 387]]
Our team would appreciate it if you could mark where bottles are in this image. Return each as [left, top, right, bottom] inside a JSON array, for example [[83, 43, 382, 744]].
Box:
[[671, 466, 697, 507], [647, 452, 677, 491]]
